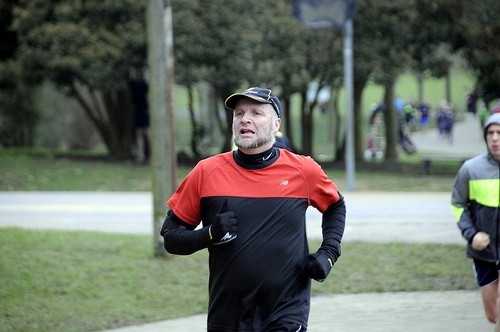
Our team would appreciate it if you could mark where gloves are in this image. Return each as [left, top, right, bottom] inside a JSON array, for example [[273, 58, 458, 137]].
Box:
[[303, 252, 333, 281], [210, 198, 238, 242], [472, 234, 491, 251]]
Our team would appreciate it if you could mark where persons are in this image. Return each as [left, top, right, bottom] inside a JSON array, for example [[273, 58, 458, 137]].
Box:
[[467, 82, 478, 114], [370, 98, 456, 145], [159, 85, 347, 332], [450, 113, 500, 331]]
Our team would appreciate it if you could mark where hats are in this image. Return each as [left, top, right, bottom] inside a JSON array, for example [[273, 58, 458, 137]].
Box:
[[224, 86, 281, 119], [482, 106, 500, 132]]
